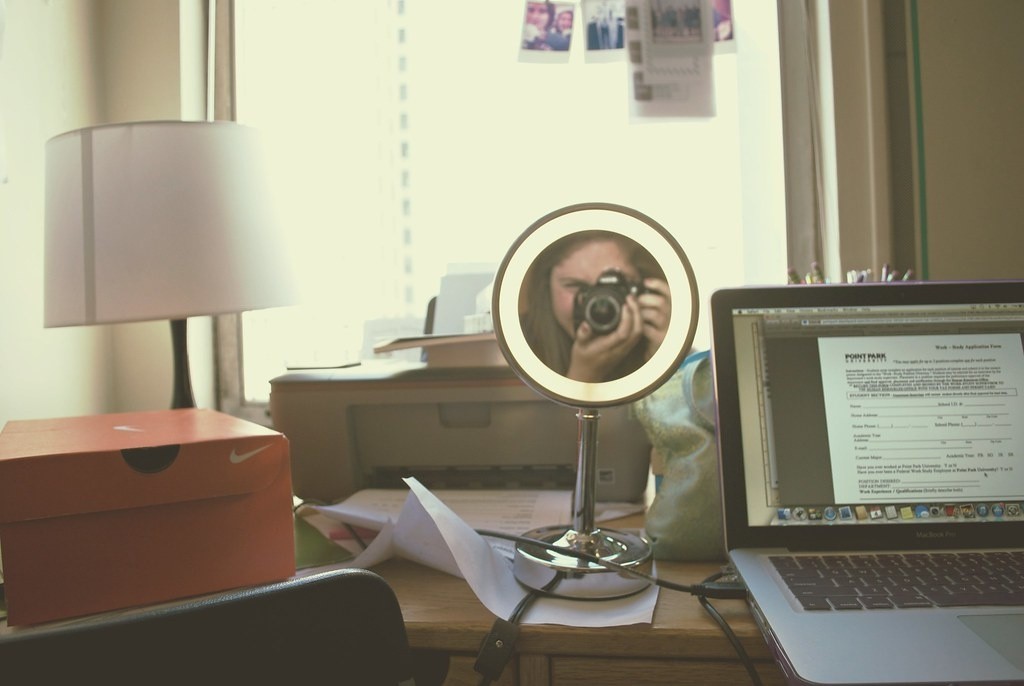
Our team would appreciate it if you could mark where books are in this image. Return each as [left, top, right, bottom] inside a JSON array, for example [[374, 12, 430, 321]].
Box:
[[370, 331, 507, 368]]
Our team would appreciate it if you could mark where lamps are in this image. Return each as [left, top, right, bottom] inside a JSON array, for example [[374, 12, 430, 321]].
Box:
[[39, 120, 290, 411]]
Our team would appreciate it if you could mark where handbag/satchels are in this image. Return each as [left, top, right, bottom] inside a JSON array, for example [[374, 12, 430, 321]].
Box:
[[628, 349, 734, 563]]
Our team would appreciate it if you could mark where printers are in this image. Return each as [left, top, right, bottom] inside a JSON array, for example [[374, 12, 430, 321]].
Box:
[[269, 332, 652, 510]]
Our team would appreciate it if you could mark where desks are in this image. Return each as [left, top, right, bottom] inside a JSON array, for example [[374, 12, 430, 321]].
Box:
[[270, 359, 651, 503], [306, 489, 785, 686]]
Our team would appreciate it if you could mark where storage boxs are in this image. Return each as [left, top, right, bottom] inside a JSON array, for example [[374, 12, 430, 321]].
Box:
[[0, 405, 294, 625]]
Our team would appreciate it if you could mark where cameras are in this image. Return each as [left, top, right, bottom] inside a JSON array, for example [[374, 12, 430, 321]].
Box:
[[574, 268, 651, 339]]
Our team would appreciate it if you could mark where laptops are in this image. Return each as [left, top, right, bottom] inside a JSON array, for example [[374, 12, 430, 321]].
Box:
[[711, 279, 1024, 686]]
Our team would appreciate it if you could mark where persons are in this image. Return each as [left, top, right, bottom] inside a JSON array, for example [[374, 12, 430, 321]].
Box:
[[519, 229, 673, 384], [652, 4, 700, 40], [522, 0, 576, 53], [588, 14, 624, 51]]
